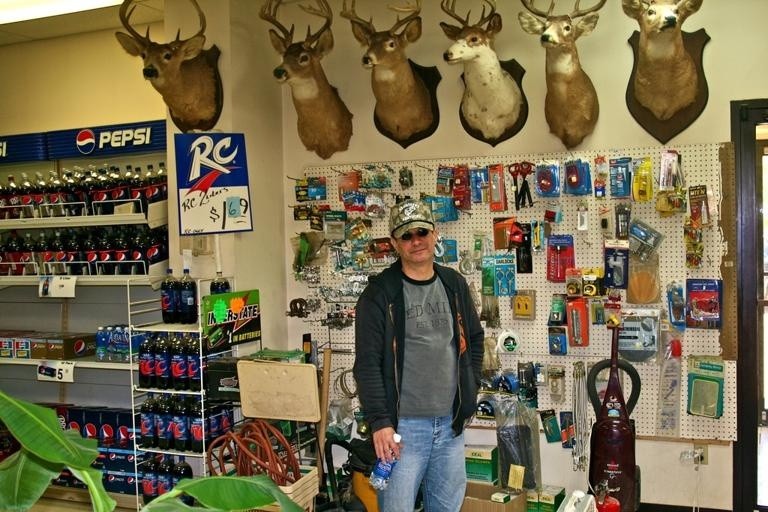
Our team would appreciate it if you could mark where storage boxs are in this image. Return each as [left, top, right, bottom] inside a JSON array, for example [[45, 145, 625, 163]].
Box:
[[462, 477, 529, 512]]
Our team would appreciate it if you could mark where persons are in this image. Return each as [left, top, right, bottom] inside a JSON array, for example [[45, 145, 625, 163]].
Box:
[[353, 198, 487, 512]]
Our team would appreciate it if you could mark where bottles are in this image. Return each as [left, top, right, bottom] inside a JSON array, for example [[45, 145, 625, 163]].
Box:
[[94, 326, 130, 364], [366, 433, 401, 491], [0, 162, 167, 274], [139, 268, 234, 508]]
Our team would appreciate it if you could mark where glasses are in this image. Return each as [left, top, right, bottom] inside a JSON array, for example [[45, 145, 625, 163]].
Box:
[[398, 229, 429, 241]]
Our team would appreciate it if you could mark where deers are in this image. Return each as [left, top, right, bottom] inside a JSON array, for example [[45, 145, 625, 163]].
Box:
[[517, 0, 607, 150], [114, 0, 216, 126], [257, 0, 354, 160], [439, 0, 525, 141], [621, 0, 703, 123], [339, 0, 434, 141]]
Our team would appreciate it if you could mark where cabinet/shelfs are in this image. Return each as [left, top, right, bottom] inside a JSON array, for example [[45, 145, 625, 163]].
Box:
[[124, 276, 245, 512], [0, 197, 170, 511]]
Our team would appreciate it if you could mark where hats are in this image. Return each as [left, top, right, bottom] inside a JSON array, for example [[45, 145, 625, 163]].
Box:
[[388, 199, 435, 239]]
